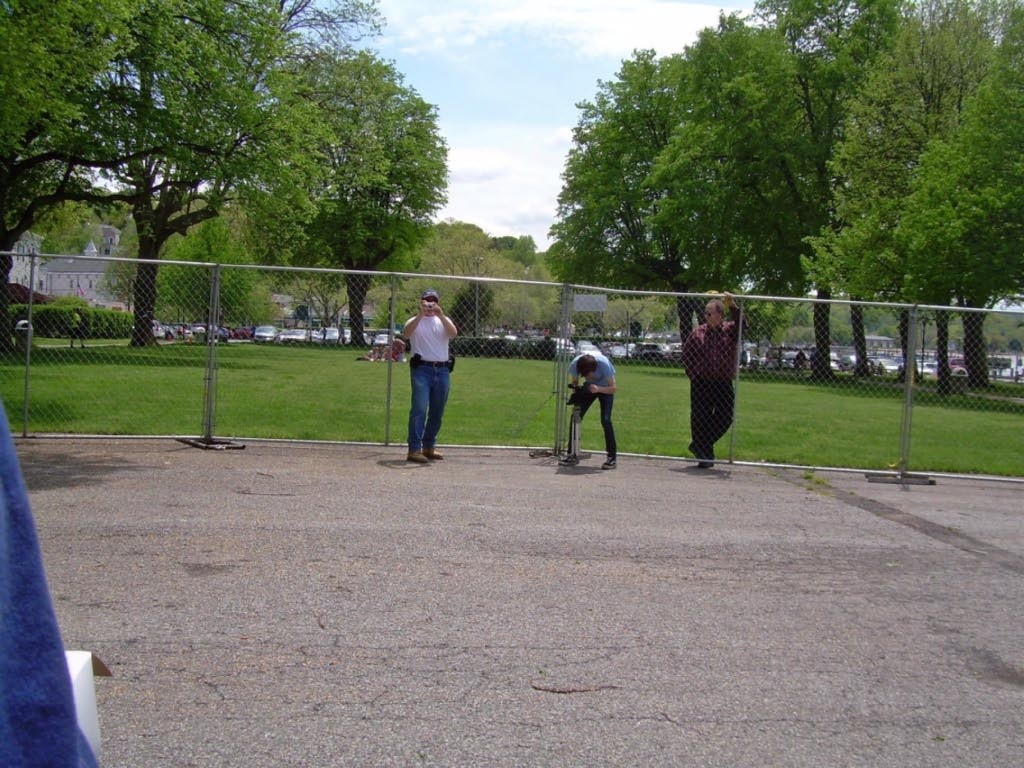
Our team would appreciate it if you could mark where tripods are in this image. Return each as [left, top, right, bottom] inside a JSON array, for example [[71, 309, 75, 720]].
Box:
[[569, 407, 581, 464]]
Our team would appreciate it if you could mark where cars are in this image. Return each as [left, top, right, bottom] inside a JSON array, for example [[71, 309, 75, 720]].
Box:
[[152, 318, 411, 353], [738, 339, 970, 380], [483, 325, 684, 363]]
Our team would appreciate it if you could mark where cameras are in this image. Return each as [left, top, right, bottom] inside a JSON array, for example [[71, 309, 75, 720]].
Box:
[[427, 303, 434, 309]]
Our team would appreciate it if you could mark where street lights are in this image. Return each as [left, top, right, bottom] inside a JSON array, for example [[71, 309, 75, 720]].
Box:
[[474, 257, 484, 338], [521, 267, 530, 331]]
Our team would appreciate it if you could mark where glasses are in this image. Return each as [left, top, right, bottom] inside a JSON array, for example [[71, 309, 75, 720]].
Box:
[[704, 311, 718, 316]]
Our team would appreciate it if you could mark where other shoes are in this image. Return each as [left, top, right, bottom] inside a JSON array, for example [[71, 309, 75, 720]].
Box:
[[408, 451, 428, 462], [601, 457, 616, 469], [422, 448, 443, 459], [688, 442, 714, 463], [558, 456, 579, 466]]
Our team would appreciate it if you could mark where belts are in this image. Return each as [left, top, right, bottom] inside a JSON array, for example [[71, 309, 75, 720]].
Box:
[[418, 360, 451, 368]]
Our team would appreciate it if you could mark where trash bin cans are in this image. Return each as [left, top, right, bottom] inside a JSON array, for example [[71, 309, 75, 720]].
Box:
[[14, 320, 33, 349], [950, 368, 969, 396], [194, 326, 206, 343]]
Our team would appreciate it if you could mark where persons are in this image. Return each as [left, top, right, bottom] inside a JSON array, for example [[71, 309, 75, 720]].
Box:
[[558, 352, 617, 469], [809, 347, 816, 370], [337, 325, 344, 344], [250, 325, 256, 338], [794, 350, 807, 377], [404, 289, 457, 463], [322, 328, 327, 339], [870, 362, 884, 374], [71, 308, 85, 348], [681, 292, 747, 467], [368, 338, 405, 361]]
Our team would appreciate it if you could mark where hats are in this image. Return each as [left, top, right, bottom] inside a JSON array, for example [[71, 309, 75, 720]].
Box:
[[422, 289, 439, 302]]
